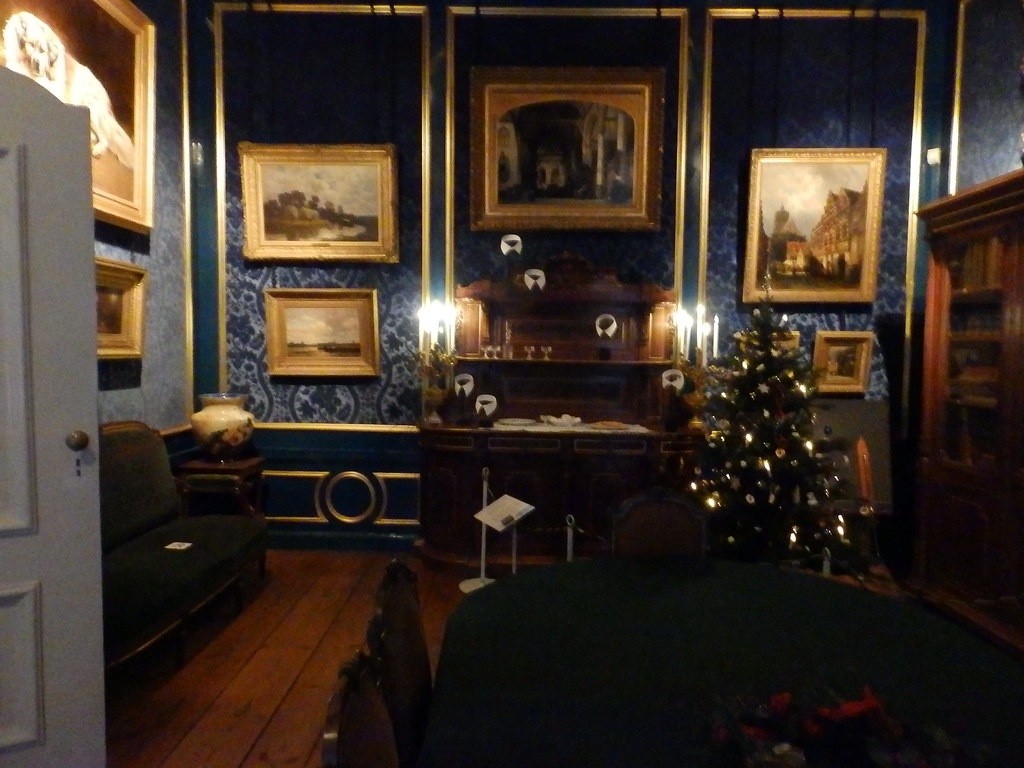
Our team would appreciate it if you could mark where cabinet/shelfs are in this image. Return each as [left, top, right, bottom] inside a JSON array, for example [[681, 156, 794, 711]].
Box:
[[909, 167, 1024, 663], [421, 275, 676, 553]]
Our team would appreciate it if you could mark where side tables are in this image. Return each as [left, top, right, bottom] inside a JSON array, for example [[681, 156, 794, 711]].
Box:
[[179, 454, 266, 517]]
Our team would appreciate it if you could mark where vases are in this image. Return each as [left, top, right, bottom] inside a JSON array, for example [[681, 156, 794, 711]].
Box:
[[186, 392, 258, 462]]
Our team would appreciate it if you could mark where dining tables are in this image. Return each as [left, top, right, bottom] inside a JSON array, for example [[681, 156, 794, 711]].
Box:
[[413, 557, 1024, 768]]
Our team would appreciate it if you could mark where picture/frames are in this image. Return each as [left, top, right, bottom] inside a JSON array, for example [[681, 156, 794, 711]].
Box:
[[0, 0, 157, 230], [809, 330, 874, 393], [94, 256, 147, 360], [237, 140, 401, 264], [741, 146, 887, 305], [467, 66, 666, 231], [262, 287, 381, 377]]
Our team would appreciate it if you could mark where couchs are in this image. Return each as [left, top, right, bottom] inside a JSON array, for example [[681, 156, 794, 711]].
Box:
[[100, 420, 271, 674]]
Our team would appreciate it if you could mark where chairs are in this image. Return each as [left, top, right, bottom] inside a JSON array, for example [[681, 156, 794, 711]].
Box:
[[367, 557, 431, 765], [321, 646, 401, 768]]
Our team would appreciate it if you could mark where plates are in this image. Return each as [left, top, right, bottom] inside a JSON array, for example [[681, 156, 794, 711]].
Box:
[[549, 417, 581, 426], [590, 421, 629, 429], [498, 418, 537, 425]]
